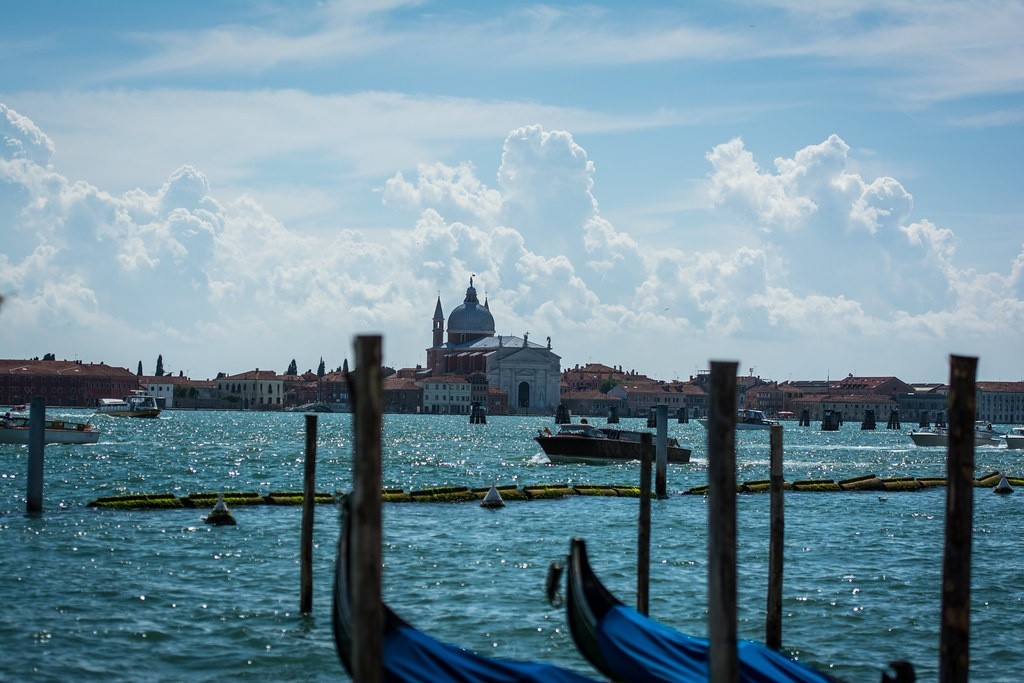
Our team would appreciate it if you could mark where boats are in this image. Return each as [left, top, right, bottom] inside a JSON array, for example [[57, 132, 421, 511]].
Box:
[[0, 404, 102, 445], [92, 389, 162, 418], [905, 423, 1004, 447], [544, 536, 918, 682], [697, 409, 779, 431], [533, 422, 693, 465], [325, 502, 615, 683], [999, 428, 1024, 449]]
